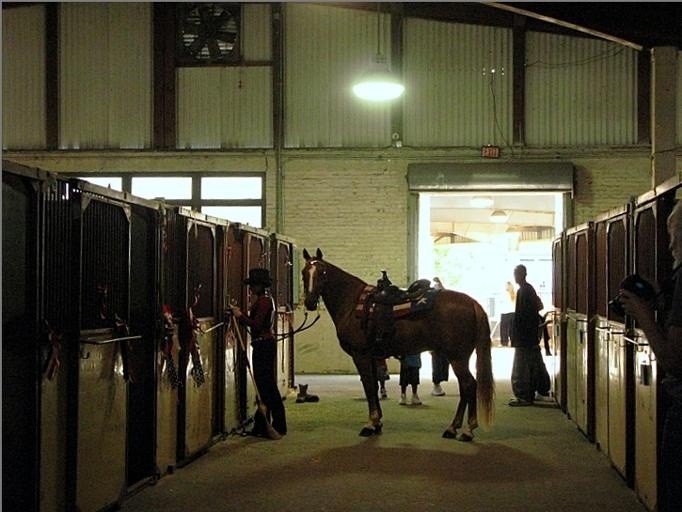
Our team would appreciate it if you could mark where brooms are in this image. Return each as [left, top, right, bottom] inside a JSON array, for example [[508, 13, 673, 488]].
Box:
[[231, 313, 282, 439]]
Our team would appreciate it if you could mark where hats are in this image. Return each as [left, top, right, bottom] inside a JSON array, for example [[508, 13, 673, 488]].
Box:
[[244, 267, 272, 286]]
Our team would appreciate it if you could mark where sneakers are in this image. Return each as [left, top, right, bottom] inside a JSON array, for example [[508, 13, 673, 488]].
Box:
[[507, 398, 533, 407], [378, 390, 446, 405]]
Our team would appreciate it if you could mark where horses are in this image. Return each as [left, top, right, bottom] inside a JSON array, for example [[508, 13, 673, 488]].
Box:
[[300, 247, 497, 442]]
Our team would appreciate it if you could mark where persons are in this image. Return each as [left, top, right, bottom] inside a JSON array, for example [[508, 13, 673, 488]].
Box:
[[533, 295, 557, 404], [392, 346, 424, 407], [376, 359, 393, 398], [507, 263, 539, 408], [430, 350, 452, 395], [226, 266, 288, 436], [617, 202, 682, 510], [433, 277, 445, 290]]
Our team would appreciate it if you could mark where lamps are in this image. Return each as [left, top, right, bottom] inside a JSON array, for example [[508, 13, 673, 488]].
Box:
[[351, 3, 407, 103]]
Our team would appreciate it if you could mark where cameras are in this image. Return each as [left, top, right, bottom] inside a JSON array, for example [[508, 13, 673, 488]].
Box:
[[606, 273, 655, 316]]
